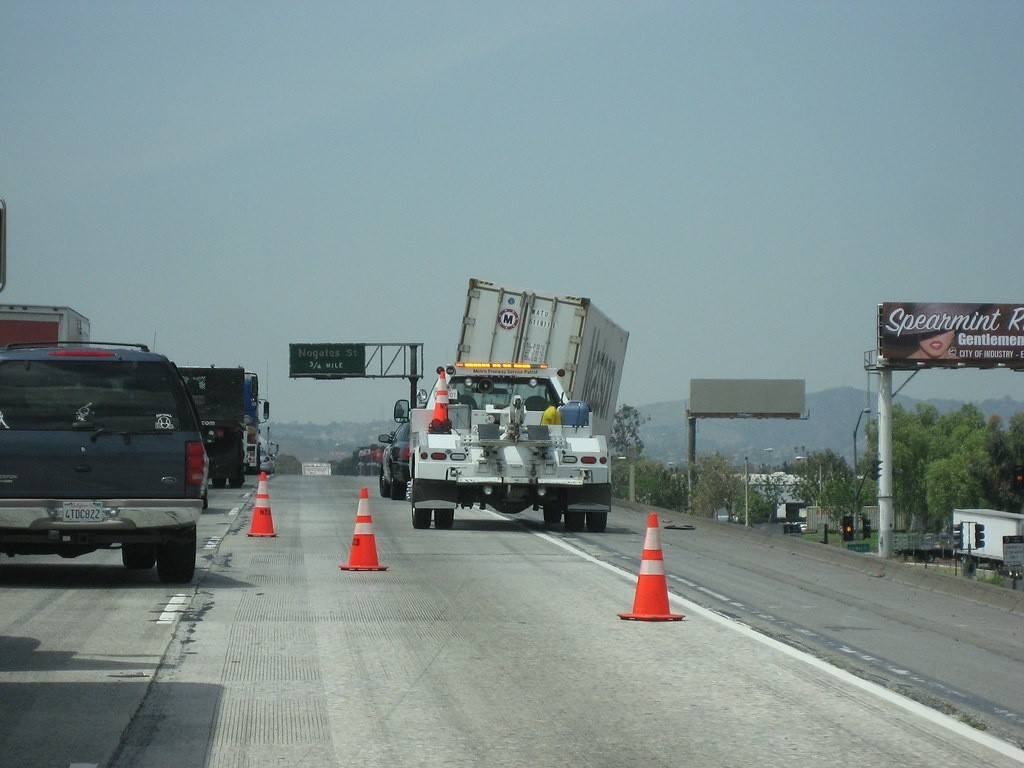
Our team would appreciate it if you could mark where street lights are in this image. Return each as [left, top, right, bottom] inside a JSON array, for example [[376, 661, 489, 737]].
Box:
[[796, 455, 822, 497], [744, 448, 774, 527], [853, 408, 871, 499]]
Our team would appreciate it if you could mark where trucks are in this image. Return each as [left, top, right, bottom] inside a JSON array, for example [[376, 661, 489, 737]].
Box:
[[455, 278, 630, 449], [175, 365, 252, 489], [243, 371, 270, 474]]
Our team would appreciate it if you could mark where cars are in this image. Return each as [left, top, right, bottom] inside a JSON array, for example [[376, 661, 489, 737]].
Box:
[[378, 420, 409, 500], [784, 521, 809, 534], [259, 449, 277, 476]]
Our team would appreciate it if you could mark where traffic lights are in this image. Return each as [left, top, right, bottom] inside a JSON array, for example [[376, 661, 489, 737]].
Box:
[[953, 523, 963, 551], [869, 458, 883, 482], [974, 523, 985, 548]]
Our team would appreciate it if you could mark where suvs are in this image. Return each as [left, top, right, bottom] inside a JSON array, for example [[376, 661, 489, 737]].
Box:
[[0, 340, 210, 584]]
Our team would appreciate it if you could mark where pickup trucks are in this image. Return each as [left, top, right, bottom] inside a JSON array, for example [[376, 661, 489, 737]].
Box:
[[393, 361, 613, 532]]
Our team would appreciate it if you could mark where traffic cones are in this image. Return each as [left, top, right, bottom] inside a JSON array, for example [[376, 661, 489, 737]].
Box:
[[246, 472, 278, 537], [337, 487, 390, 571], [431, 370, 450, 429], [617, 512, 686, 621]]
